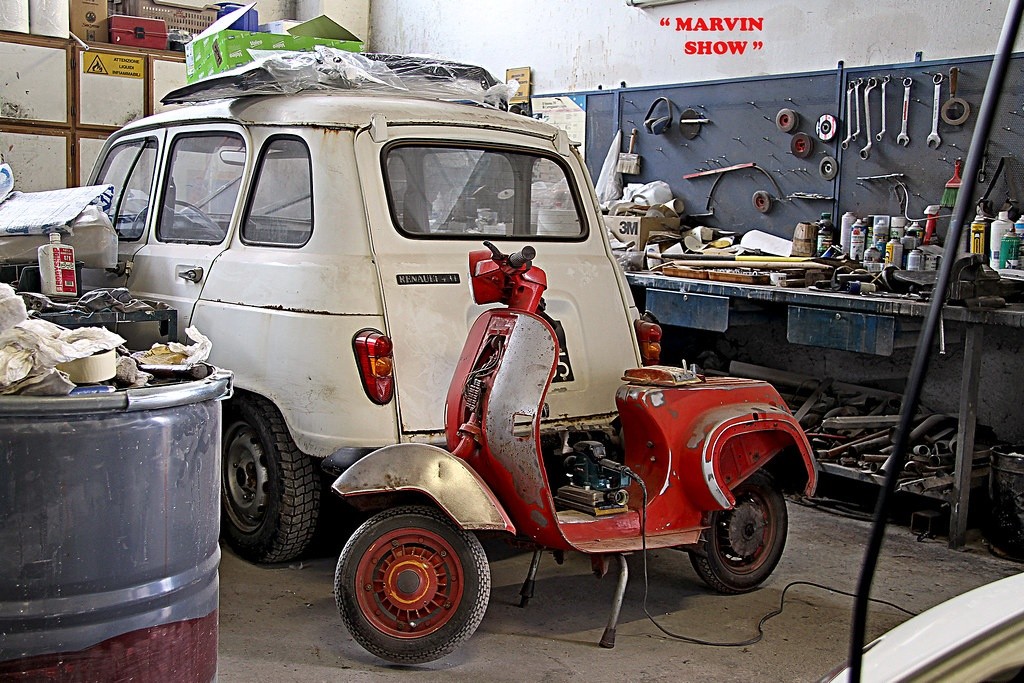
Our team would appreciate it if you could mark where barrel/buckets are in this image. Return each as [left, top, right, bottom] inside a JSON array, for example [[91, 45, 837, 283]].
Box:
[[0, 362, 234, 683]]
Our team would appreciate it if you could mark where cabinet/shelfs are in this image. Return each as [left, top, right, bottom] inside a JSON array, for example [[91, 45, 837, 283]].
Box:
[[0, 30, 188, 193]]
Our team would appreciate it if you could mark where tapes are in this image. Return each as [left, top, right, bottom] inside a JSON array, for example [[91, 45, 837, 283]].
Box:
[[56, 348, 116, 384]]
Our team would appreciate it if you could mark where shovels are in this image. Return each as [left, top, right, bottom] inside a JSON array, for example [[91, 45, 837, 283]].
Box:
[[938, 67, 963, 133]]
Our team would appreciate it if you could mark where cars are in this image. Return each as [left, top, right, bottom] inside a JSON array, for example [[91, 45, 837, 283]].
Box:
[[71, 91, 662, 568]]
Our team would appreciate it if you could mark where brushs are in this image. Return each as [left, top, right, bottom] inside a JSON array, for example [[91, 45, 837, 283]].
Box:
[[940, 159, 962, 208], [616, 128, 640, 175]]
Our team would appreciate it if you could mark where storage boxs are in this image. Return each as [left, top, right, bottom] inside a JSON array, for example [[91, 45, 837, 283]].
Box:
[[536, 208, 682, 252], [68, 0, 258, 53], [184, 1, 366, 85]]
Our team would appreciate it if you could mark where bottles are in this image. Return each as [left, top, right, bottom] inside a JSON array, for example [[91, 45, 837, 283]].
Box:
[[999, 229, 1021, 269], [816, 213, 835, 256], [840, 211, 924, 271], [1014, 215, 1024, 269], [990, 211, 1013, 270], [37, 232, 76, 299], [971, 215, 990, 257]]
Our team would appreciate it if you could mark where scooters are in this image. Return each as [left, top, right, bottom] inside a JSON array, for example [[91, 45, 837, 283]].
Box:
[[330, 244, 819, 665]]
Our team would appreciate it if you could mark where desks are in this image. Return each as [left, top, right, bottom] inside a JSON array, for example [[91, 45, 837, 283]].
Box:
[[622, 271, 1024, 552]]
